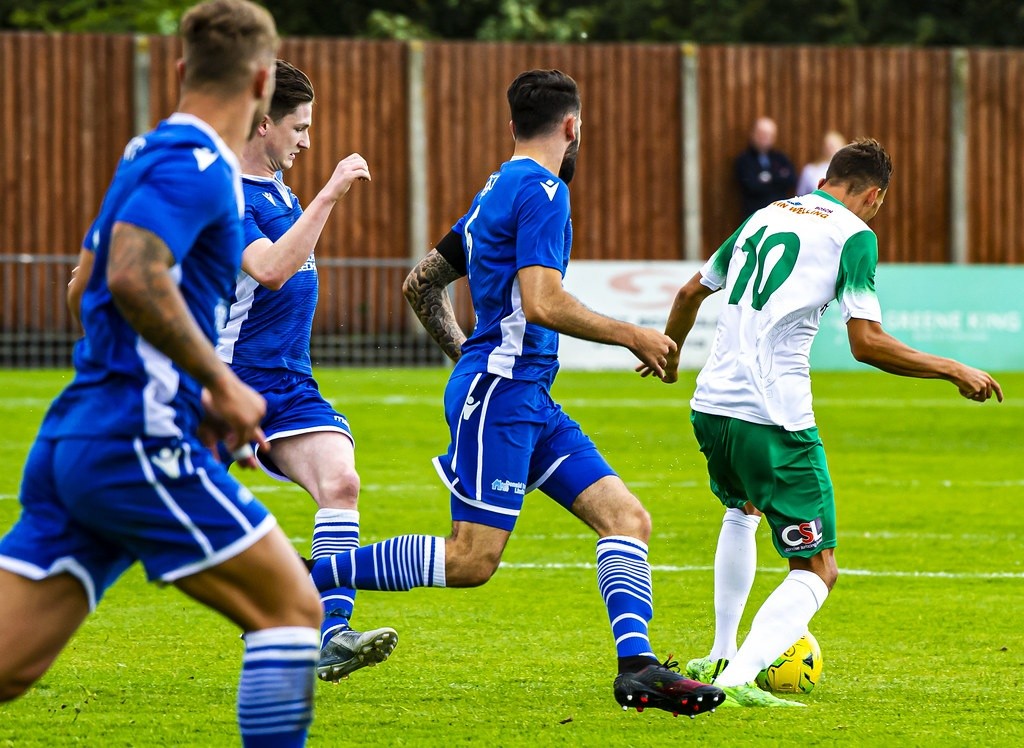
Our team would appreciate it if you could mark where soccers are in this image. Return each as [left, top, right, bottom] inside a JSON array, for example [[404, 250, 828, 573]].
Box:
[[753, 627, 824, 695]]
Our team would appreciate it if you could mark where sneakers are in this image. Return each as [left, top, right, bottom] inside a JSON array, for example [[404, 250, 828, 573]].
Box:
[[316, 627, 399, 684], [711, 679, 805, 708], [685, 657, 728, 682], [613, 654, 726, 719]]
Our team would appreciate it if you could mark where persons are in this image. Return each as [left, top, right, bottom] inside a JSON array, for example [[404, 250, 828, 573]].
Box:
[[731, 118, 798, 225], [0, 0, 323, 748], [797, 131, 845, 196], [636, 140, 1003, 709], [301, 69, 726, 718], [208, 59, 399, 683]]
